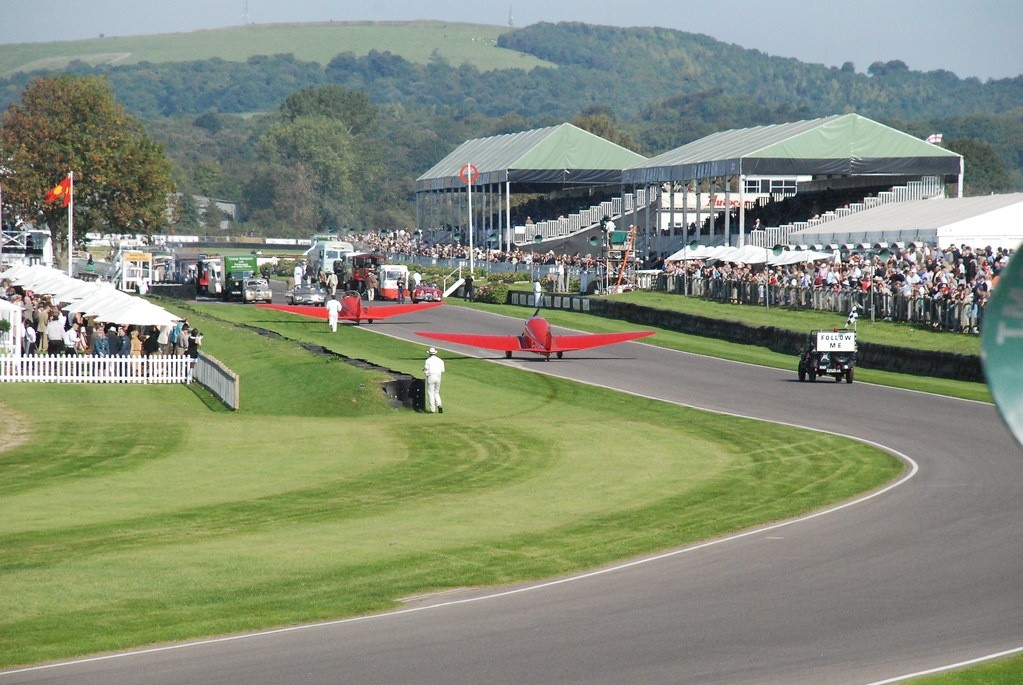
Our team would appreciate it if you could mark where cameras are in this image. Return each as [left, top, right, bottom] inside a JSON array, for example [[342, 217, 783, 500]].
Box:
[[200, 332, 203, 337]]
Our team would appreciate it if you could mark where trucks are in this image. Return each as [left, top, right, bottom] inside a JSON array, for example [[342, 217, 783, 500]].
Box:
[[194, 259, 220, 296], [207, 262, 221, 297], [315, 241, 354, 278], [220, 254, 258, 302]]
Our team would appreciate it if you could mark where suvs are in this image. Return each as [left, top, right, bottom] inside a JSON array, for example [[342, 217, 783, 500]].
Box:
[[798, 327, 857, 383]]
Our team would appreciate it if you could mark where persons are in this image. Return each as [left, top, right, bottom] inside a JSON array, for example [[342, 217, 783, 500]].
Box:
[[293, 260, 377, 301], [636, 187, 893, 233], [464, 275, 473, 302], [834, 326, 838, 332], [326, 295, 341, 333], [872, 242, 1015, 336], [424, 348, 445, 413], [0, 279, 204, 383], [340, 229, 595, 266], [534, 279, 541, 308], [555, 260, 570, 292], [580, 264, 590, 293], [413, 270, 422, 285], [444, 195, 611, 231], [137, 276, 148, 294], [262, 268, 270, 284], [663, 254, 872, 315], [605, 217, 616, 239], [397, 277, 405, 304]]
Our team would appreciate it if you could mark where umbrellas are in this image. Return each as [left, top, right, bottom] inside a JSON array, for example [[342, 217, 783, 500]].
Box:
[[0, 262, 183, 326], [667, 244, 833, 267]]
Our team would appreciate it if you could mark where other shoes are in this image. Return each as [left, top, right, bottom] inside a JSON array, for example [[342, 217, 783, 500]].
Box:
[[438, 406, 443, 413]]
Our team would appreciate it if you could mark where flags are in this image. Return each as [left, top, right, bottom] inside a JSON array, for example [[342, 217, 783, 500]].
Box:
[[845, 305, 858, 327], [45, 178, 70, 207]]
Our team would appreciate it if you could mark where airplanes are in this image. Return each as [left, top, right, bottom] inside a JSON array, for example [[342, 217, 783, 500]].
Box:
[[415, 318, 656, 362], [255, 290, 446, 326]]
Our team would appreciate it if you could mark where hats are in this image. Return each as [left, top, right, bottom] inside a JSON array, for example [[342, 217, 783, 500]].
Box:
[[107, 326, 118, 333], [330, 270, 335, 273], [427, 347, 438, 354]]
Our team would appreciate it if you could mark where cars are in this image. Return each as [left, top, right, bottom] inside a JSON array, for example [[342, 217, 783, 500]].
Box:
[[377, 269, 408, 301], [410, 283, 443, 304], [284, 284, 326, 307], [353, 254, 385, 293], [242, 278, 272, 304], [341, 252, 367, 291]]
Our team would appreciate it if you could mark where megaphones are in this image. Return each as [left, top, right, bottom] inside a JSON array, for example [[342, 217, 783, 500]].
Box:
[[686, 240, 699, 251], [328, 227, 367, 235], [414, 231, 420, 239], [587, 235, 599, 246], [533, 234, 543, 243], [453, 232, 461, 241], [766, 244, 782, 256], [488, 232, 497, 241], [381, 229, 388, 236]]
